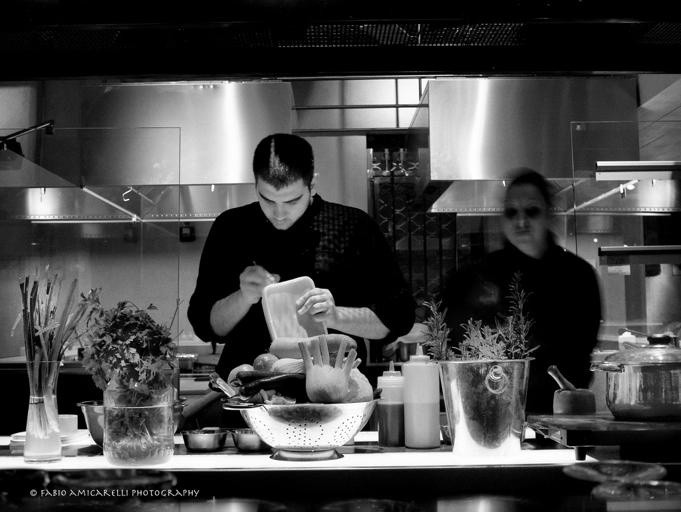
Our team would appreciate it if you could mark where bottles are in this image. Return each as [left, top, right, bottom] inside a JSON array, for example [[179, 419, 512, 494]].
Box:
[[402, 343, 443, 450], [375, 360, 404, 448]]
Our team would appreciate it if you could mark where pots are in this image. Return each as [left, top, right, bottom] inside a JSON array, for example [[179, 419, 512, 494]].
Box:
[[591, 332, 679, 419]]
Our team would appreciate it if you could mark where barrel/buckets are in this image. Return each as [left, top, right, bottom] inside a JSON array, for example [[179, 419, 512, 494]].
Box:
[[438, 359, 531, 454]]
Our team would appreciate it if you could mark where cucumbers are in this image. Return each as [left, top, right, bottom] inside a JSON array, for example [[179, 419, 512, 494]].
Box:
[[230, 371, 311, 404]]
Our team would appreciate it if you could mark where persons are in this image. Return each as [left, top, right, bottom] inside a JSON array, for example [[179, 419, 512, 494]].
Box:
[[189, 133, 414, 428], [443, 168, 604, 431]]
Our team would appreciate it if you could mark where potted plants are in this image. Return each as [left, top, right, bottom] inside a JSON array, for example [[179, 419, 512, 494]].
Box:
[[78, 289, 176, 465], [11, 270, 97, 462]]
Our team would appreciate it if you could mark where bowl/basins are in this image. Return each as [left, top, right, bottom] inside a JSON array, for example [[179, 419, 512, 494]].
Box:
[[184, 429, 227, 451], [230, 427, 273, 452], [76, 399, 188, 455], [552, 388, 596, 413], [223, 399, 379, 461]]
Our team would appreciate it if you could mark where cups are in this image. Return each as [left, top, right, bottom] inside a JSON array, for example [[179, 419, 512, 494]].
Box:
[[58, 413, 78, 452]]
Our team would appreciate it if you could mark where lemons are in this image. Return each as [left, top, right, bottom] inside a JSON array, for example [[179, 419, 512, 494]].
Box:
[[253, 354, 279, 371]]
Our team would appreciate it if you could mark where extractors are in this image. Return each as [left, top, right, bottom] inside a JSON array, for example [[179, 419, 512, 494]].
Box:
[[0, 80, 678, 236]]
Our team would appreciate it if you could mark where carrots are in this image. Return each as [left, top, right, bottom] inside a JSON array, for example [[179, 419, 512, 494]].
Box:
[[270, 334, 357, 359]]
